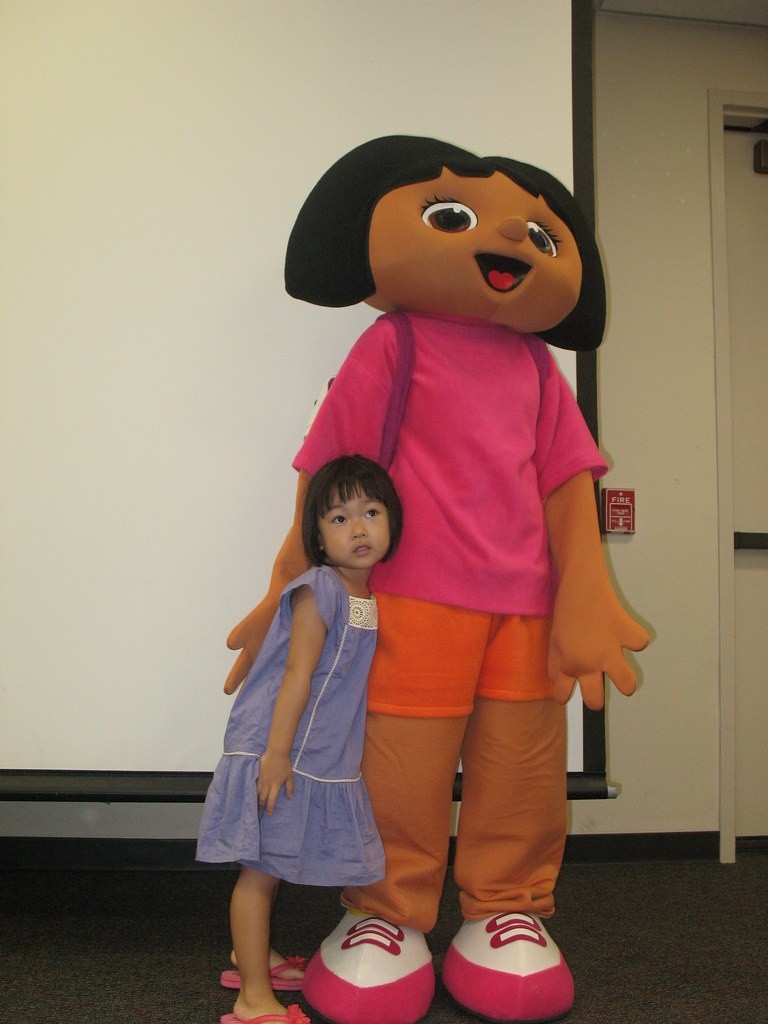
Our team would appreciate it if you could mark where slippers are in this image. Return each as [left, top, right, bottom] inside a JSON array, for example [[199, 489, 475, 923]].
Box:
[[221, 956, 309, 989], [220, 1003, 311, 1024]]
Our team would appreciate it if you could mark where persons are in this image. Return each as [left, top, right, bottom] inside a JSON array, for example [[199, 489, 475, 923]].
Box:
[[193, 456, 403, 1024]]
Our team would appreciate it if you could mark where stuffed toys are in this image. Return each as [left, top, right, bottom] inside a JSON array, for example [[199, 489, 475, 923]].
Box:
[[223, 136, 649, 1024]]
